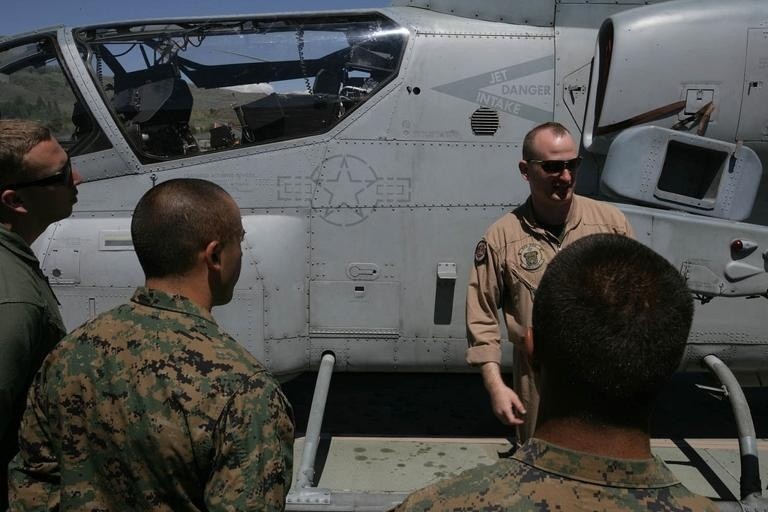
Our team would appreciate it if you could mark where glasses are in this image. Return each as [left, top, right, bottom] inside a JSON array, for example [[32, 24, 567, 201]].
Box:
[[4, 151, 71, 190], [528, 156, 583, 174]]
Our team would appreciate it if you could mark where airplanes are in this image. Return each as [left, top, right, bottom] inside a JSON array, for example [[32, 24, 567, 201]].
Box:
[[1, 1, 767, 390]]
